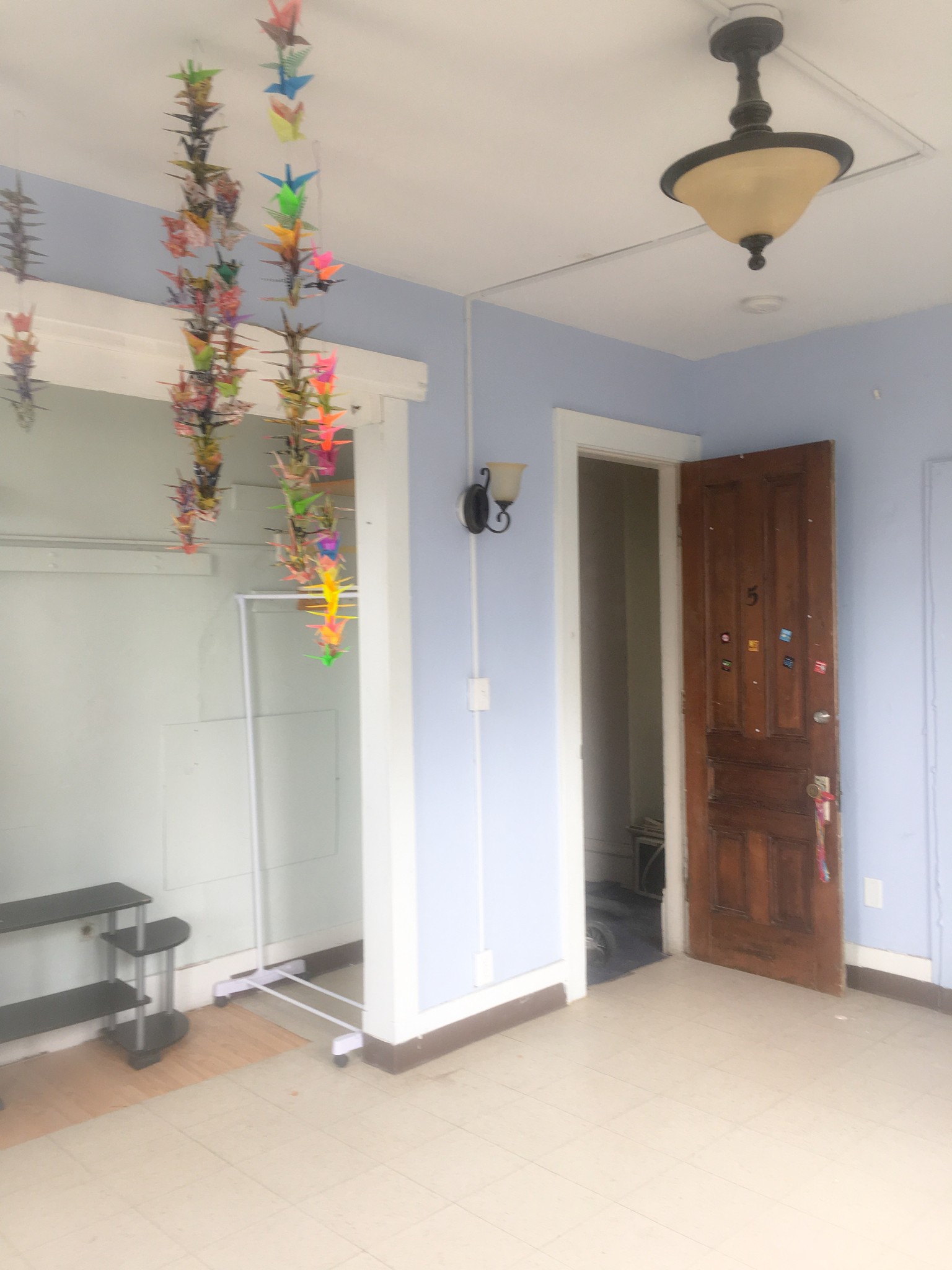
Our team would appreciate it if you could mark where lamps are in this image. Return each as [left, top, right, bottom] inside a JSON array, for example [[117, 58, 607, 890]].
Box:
[[457, 461, 528, 534], [661, 3, 854, 269]]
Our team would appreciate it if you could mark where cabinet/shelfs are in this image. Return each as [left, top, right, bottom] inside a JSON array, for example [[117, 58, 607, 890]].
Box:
[[0, 882, 191, 1070]]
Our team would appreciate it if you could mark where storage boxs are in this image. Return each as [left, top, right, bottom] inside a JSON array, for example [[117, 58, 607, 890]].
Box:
[[634, 838, 665, 900]]
